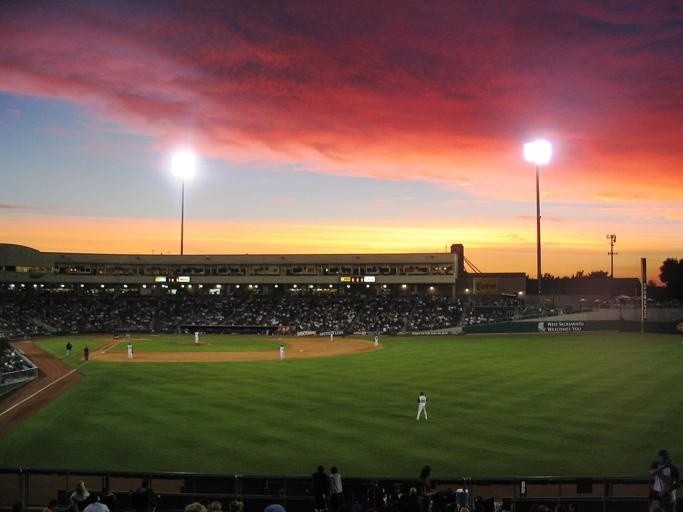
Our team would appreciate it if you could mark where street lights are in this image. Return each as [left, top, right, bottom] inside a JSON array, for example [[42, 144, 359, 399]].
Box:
[[171, 146, 197, 256], [523, 140, 553, 294]]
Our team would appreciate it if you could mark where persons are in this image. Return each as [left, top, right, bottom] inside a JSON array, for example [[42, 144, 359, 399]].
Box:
[[0, 282, 566, 343], [374, 334, 379, 347], [0, 344, 36, 386], [65, 341, 73, 355], [415, 391, 427, 421], [279, 344, 285, 361], [9, 465, 574, 512], [648, 449, 680, 512], [125, 344, 134, 359], [83, 344, 89, 362]]
